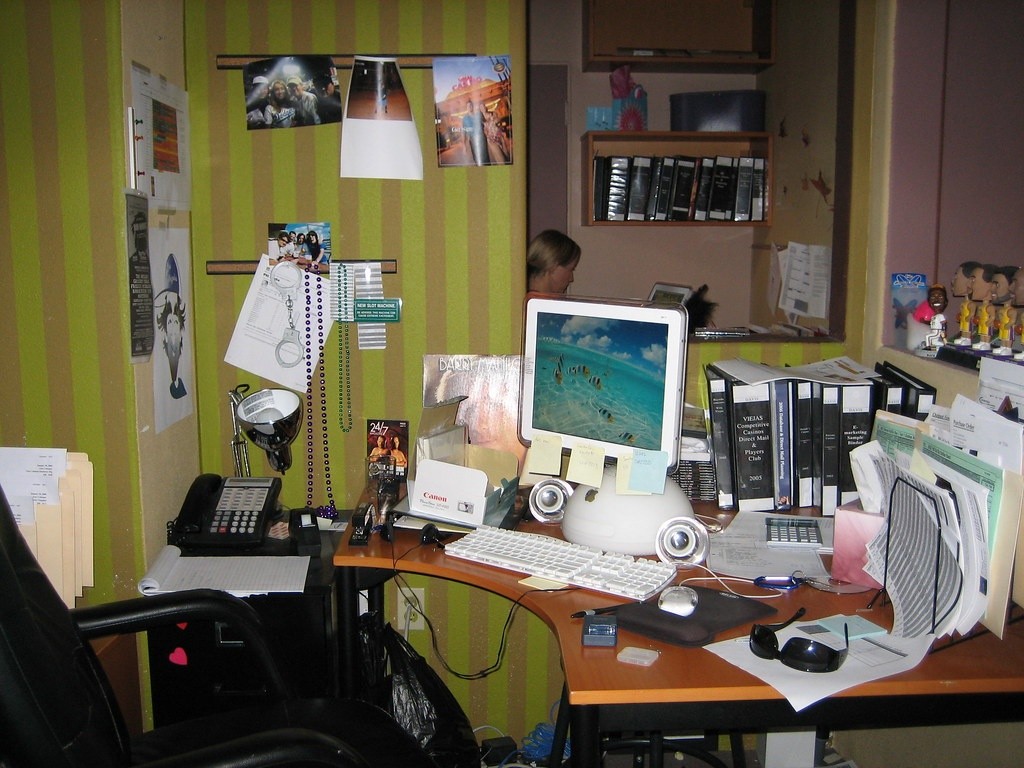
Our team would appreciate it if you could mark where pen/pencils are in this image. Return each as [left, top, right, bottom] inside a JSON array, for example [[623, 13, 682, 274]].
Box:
[[571, 604, 623, 618]]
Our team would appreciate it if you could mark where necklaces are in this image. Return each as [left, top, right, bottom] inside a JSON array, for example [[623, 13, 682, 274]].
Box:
[[306, 264, 352, 521]]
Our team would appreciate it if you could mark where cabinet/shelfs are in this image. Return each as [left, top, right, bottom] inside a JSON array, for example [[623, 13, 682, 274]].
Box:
[[580, 0, 779, 227]]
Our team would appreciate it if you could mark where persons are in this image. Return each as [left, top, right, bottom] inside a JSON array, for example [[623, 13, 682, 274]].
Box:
[[247, 72, 341, 129], [461, 101, 491, 166], [375, 82, 388, 113], [527, 228, 582, 293], [919, 284, 948, 350], [369, 436, 407, 468], [269, 231, 328, 265], [951, 262, 1024, 360]]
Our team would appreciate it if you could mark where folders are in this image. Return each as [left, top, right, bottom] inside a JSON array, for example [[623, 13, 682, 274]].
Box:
[[868, 376, 903, 414], [702, 363, 734, 510], [875, 361, 936, 422]]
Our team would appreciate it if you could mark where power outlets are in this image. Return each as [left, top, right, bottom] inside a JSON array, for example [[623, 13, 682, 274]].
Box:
[[397, 588, 424, 630]]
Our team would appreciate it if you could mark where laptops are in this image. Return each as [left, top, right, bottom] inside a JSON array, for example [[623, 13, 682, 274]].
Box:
[[649, 282, 690, 308]]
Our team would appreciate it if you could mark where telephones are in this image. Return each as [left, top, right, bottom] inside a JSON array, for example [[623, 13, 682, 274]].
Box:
[[171, 473, 283, 548]]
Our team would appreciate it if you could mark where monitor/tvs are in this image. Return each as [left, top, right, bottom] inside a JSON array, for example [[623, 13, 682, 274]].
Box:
[[516, 292, 695, 557]]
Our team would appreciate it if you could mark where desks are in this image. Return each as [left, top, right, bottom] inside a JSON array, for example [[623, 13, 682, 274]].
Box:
[[147, 508, 360, 730], [334, 481, 1024, 768]]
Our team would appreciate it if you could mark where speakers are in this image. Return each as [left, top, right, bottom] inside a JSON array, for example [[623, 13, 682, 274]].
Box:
[[528, 479, 573, 525], [655, 517, 709, 570]]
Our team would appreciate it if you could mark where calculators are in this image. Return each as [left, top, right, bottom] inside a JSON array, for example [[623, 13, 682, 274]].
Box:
[[765, 518, 823, 546]]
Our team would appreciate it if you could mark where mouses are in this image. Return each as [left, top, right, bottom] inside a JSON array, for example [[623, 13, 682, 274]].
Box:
[[658, 586, 699, 617]]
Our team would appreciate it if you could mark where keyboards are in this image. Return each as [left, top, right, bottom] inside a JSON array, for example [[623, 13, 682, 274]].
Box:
[[443, 524, 677, 602]]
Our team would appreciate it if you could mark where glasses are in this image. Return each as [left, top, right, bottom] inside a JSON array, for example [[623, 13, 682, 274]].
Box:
[[749, 607, 849, 673]]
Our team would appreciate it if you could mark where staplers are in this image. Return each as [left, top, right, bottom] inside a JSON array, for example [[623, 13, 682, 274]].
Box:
[[349, 502, 375, 545]]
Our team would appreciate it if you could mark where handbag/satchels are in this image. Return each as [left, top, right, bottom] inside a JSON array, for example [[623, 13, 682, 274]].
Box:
[[379, 622, 480, 768]]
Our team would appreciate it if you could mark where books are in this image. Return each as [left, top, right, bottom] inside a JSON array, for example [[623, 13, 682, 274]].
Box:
[[137, 546, 311, 597]]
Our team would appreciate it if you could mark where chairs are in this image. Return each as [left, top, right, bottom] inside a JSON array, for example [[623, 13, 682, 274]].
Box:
[[0, 483, 445, 767]]
